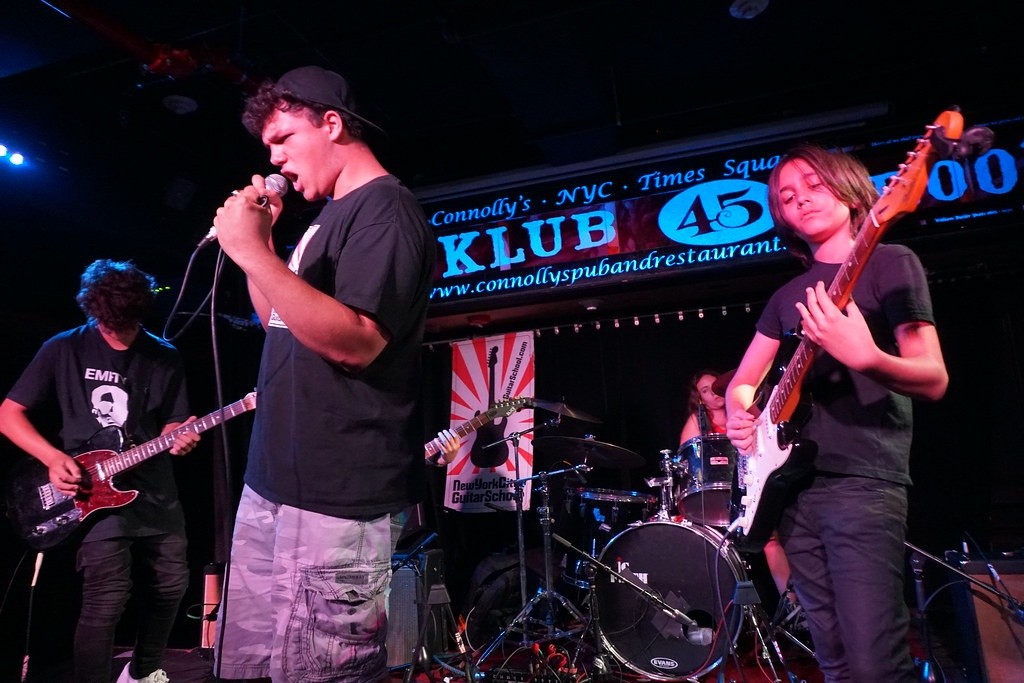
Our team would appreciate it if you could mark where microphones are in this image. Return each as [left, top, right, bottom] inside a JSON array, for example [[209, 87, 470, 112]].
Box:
[[698, 398, 715, 431], [196, 173, 288, 250], [688, 627, 715, 646]]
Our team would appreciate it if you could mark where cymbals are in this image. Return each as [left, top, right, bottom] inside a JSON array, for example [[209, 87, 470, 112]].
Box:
[[530, 432, 647, 470], [522, 396, 605, 425]]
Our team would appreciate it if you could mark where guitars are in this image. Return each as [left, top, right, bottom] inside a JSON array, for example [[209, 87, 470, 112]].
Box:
[[735, 100, 996, 556], [7, 388, 259, 552], [424, 398, 526, 461]]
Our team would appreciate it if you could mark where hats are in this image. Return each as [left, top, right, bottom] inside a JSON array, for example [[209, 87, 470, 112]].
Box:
[[273, 66, 383, 132]]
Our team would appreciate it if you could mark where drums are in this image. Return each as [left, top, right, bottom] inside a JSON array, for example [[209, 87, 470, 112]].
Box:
[[674, 432, 739, 527], [559, 484, 660, 591], [596, 518, 749, 682]]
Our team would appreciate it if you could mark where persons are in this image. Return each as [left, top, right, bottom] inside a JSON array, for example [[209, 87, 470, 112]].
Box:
[[390, 429, 460, 551], [214, 65, 434, 683], [726, 148, 949, 683], [678, 370, 807, 630], [0, 258, 201, 683]]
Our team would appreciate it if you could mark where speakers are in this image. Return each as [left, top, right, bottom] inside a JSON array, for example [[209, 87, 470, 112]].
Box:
[[384, 548, 446, 668], [934, 556, 1024, 683], [202, 562, 232, 658]]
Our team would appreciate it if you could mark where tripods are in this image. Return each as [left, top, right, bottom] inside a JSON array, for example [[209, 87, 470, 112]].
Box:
[[443, 412, 819, 683]]
